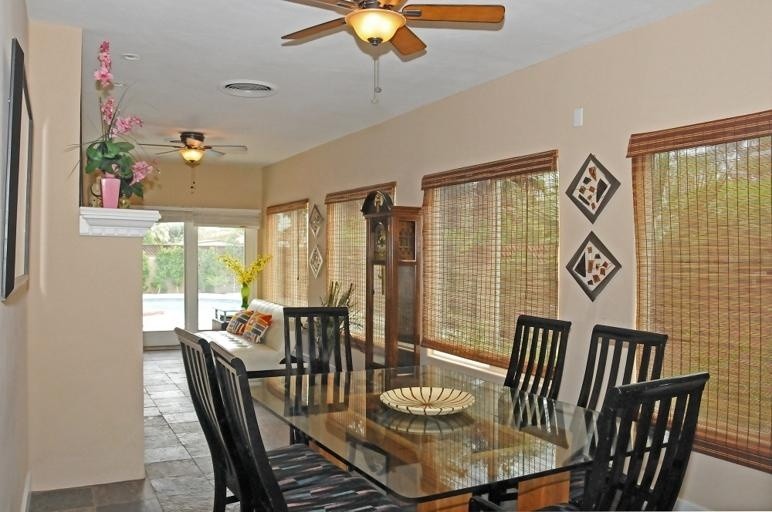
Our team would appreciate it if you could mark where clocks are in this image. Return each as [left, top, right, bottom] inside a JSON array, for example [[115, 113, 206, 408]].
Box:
[[361, 190, 424, 367]]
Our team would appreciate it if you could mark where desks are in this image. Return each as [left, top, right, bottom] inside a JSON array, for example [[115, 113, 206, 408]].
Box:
[[213, 309, 242, 331]]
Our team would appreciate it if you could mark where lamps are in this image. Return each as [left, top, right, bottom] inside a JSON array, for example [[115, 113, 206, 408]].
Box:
[[345, 0, 406, 46], [180, 132, 204, 164]]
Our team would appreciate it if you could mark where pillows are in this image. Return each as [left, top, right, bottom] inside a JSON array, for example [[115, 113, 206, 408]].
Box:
[[225, 309, 253, 334], [243, 311, 272, 342]]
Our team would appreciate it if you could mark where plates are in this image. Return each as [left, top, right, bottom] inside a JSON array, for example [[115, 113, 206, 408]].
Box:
[[380, 386, 476, 416]]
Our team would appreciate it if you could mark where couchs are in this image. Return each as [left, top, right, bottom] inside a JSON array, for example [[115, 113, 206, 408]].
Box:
[[194, 299, 309, 371]]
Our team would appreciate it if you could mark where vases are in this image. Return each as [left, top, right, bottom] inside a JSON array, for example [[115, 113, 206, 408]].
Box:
[[240, 284, 250, 308], [101, 164, 121, 208], [318, 333, 333, 372]]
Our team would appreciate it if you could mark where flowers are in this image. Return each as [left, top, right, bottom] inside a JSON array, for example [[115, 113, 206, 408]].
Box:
[[219, 255, 273, 285], [306, 281, 364, 355], [65, 41, 160, 199]]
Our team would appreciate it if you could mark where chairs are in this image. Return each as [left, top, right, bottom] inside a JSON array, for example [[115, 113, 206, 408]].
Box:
[[283, 307, 353, 445], [504, 315, 572, 426], [210, 342, 411, 512], [469, 373, 709, 512], [174, 328, 350, 512], [486, 325, 668, 505]]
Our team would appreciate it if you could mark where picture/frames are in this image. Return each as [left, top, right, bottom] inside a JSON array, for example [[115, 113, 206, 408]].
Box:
[[309, 205, 324, 238], [564, 231, 622, 303], [565, 153, 621, 225], [309, 246, 324, 280], [7, 38, 33, 304]]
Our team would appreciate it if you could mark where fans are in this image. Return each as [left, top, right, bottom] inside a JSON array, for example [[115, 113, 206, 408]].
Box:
[[138, 132, 247, 154], [281, 0, 506, 55]]
